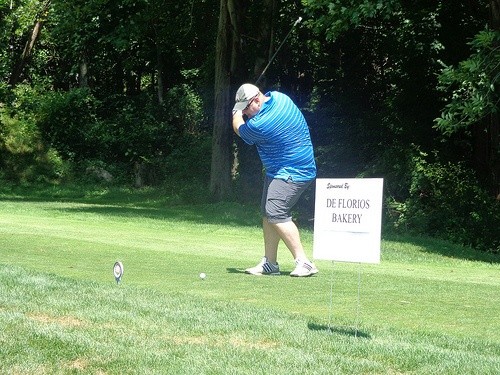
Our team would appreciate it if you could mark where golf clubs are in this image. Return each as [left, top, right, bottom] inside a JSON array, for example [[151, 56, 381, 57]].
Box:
[[253, 17, 303, 86]]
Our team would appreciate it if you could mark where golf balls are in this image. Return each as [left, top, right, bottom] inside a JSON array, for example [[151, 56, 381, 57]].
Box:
[[199, 273, 206, 278]]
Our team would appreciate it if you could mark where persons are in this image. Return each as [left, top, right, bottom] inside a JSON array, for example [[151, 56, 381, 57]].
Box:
[[233, 83, 321, 278]]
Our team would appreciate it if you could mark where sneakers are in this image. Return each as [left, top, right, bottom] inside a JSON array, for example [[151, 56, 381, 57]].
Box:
[[289, 258, 318, 277], [245, 256, 280, 276]]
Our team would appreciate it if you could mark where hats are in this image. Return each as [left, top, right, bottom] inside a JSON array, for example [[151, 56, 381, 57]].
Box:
[[232, 83, 260, 111]]
